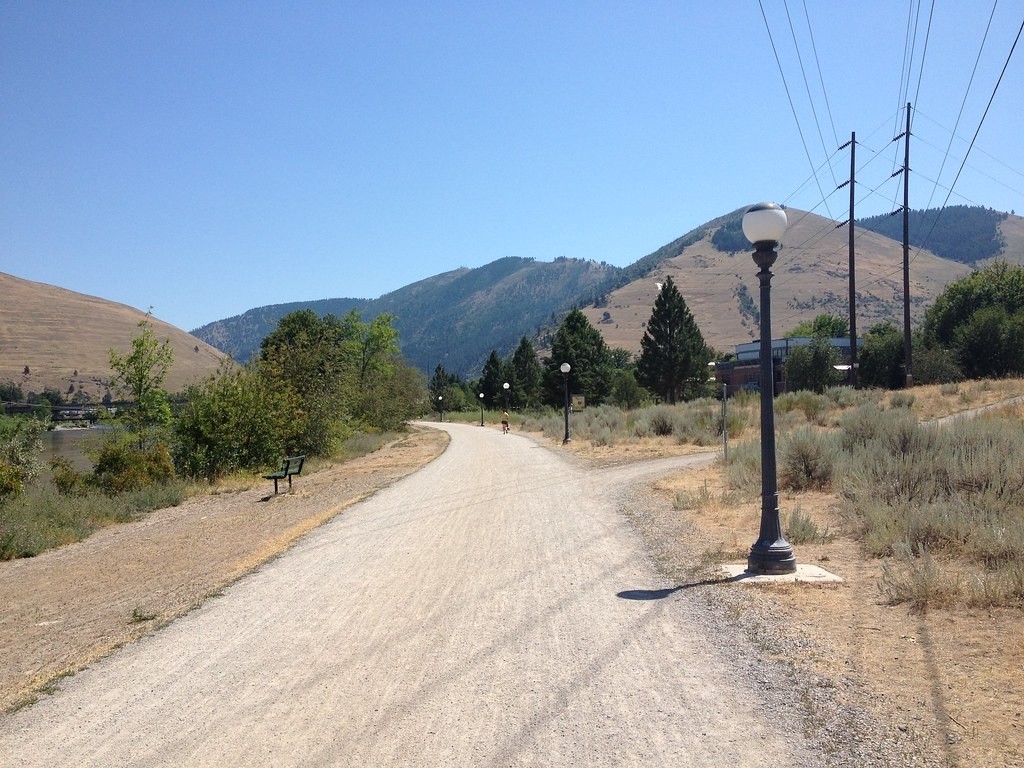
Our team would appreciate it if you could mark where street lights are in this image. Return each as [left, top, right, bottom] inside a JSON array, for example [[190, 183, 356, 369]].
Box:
[[740, 200, 799, 575], [559, 362, 573, 444], [502, 382, 510, 427], [438, 396, 443, 422], [479, 393, 485, 426]]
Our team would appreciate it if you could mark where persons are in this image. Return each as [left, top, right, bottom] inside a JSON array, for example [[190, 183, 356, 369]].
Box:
[[500, 410, 509, 434]]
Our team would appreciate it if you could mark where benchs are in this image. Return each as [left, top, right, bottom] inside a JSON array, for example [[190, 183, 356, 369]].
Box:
[[262, 454, 305, 494]]
